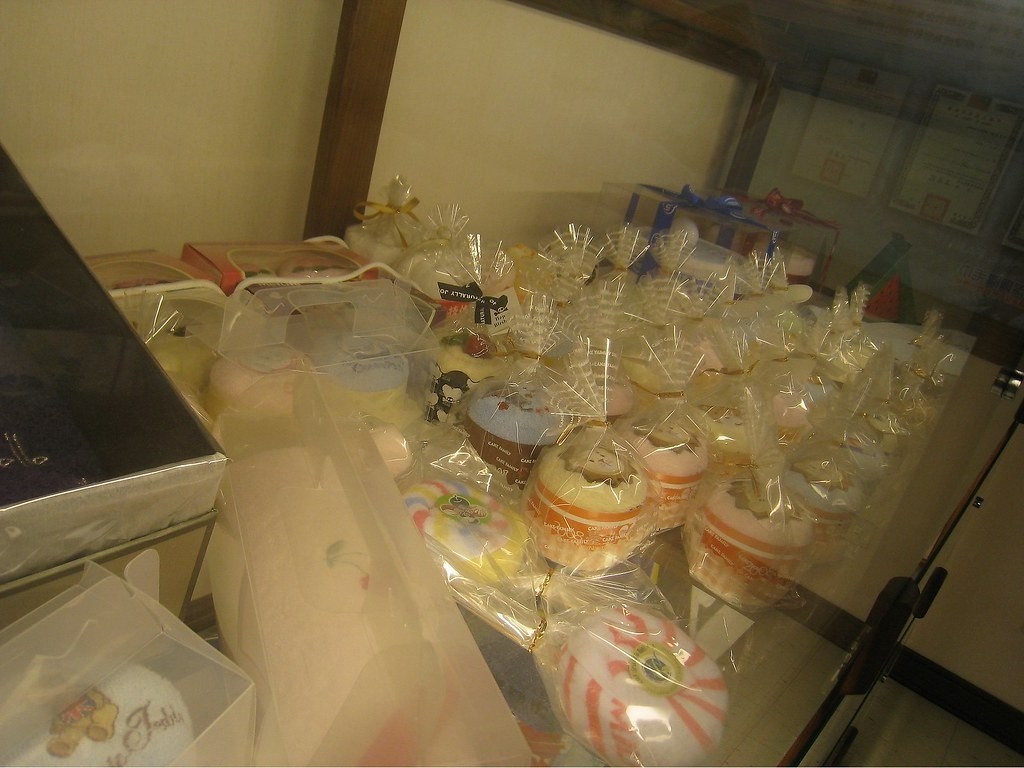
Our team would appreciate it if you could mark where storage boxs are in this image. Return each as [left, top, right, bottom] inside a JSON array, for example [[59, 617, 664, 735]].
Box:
[[693, 185, 847, 293], [601, 182, 802, 272], [81, 249, 221, 301], [180, 241, 378, 316], [185, 284, 442, 437], [206, 359, 531, 767], [0, 549, 255, 768]]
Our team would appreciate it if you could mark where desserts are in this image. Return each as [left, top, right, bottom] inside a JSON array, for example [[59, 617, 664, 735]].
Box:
[[124, 213, 906, 767]]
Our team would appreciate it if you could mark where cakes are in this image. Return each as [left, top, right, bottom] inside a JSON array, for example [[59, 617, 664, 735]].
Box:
[[0, 665, 198, 767]]
